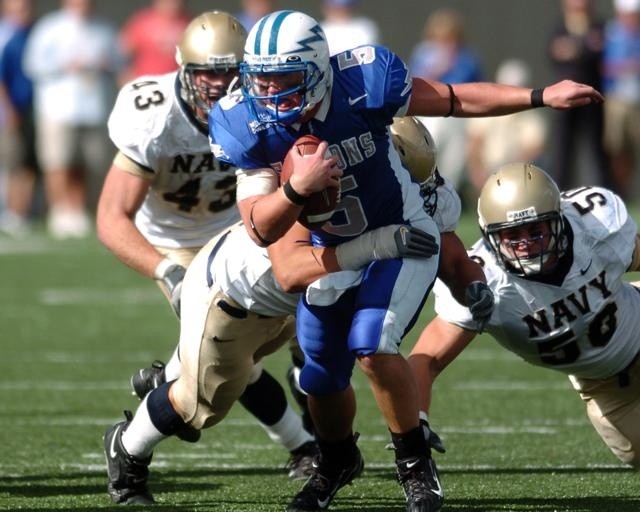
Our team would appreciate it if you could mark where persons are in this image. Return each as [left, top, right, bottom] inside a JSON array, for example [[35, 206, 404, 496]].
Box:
[[99, 116, 495, 505], [96, 8, 326, 480], [405, 161, 640, 469], [207, 10, 607, 512], [2, 0, 640, 238]]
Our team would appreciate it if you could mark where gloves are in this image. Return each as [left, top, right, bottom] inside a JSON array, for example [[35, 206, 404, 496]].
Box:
[[465, 280, 495, 335], [153, 257, 186, 318], [335, 225, 438, 272]]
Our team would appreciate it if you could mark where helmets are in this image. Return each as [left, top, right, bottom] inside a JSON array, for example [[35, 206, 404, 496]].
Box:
[[176, 10, 247, 115], [476, 162, 564, 278], [388, 115, 441, 215], [242, 11, 328, 123]]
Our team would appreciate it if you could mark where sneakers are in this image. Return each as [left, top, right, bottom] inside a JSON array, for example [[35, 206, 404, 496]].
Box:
[[103, 422, 157, 510], [395, 445, 444, 511], [384, 411, 445, 456], [287, 434, 365, 512], [130, 359, 165, 401]]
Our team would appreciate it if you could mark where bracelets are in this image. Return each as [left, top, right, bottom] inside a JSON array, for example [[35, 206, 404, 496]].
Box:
[[282, 179, 309, 206], [532, 87, 544, 107]]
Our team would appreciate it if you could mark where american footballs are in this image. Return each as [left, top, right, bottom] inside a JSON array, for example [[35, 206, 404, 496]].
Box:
[[280, 135, 341, 233]]
